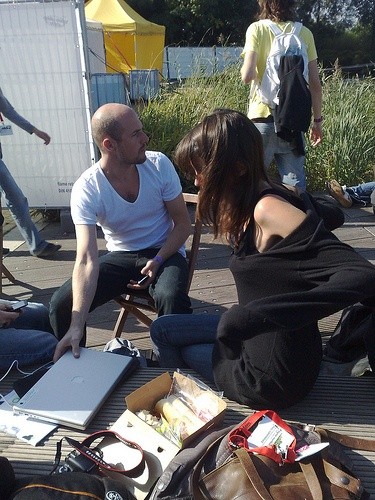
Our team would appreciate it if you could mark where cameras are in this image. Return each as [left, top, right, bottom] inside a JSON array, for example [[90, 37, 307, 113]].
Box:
[[58, 450, 97, 474]]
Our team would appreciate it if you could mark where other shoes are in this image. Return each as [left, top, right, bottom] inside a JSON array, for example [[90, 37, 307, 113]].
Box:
[[37, 243, 61, 257], [326, 180, 353, 209], [3, 248, 9, 254]]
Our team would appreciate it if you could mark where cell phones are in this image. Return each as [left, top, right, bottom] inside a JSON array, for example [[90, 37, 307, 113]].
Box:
[[5, 300, 28, 311], [138, 276, 148, 284]]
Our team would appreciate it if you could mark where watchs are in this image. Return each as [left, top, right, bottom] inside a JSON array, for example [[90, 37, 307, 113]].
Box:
[[312, 116, 323, 122]]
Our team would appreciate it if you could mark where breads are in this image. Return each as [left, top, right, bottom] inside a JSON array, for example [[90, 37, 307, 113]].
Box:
[[155, 394, 206, 436]]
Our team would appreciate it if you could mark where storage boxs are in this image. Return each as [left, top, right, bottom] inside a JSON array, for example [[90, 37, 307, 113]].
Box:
[[94, 371, 228, 500]]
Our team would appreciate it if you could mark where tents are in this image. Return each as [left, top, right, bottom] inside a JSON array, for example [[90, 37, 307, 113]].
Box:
[[75, 0, 165, 90]]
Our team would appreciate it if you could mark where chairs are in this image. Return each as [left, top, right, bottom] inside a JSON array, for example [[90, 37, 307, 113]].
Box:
[[107, 192, 206, 346]]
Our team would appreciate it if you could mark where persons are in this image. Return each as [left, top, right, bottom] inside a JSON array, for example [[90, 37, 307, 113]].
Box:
[[239, 0, 322, 192], [0, 85, 62, 257], [150, 110, 375, 414], [328, 179, 375, 208], [0, 299, 59, 370], [49, 103, 193, 363]]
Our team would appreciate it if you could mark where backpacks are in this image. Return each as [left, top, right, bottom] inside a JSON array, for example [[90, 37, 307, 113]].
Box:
[[253, 18, 310, 111]]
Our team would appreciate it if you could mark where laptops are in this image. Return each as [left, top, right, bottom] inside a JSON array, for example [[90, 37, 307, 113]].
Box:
[[12, 347, 133, 431]]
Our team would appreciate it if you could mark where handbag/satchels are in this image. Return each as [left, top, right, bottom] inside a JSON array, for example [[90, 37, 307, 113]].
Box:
[[194, 450, 364, 500]]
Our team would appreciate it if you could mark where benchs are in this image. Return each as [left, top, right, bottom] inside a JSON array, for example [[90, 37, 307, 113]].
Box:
[[0, 360, 375, 500]]
